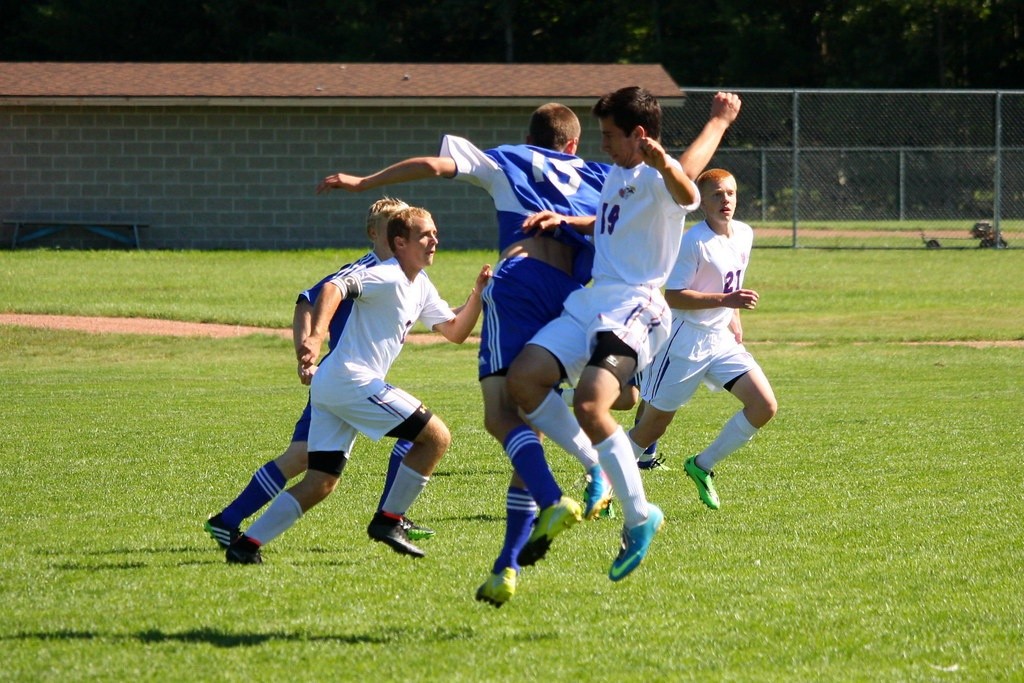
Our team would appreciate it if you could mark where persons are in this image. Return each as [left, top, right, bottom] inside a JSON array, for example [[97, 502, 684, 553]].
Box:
[[203, 86, 777, 609]]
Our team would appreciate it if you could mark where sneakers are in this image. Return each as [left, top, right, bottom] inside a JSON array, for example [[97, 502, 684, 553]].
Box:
[[584, 461, 614, 521], [609, 502, 664, 582], [202, 513, 241, 552], [475, 567, 517, 610], [400, 514, 435, 539], [517, 495, 583, 567], [225, 533, 269, 563], [636, 441, 667, 469], [583, 482, 607, 515], [368, 508, 425, 557], [682, 455, 721, 510]]
